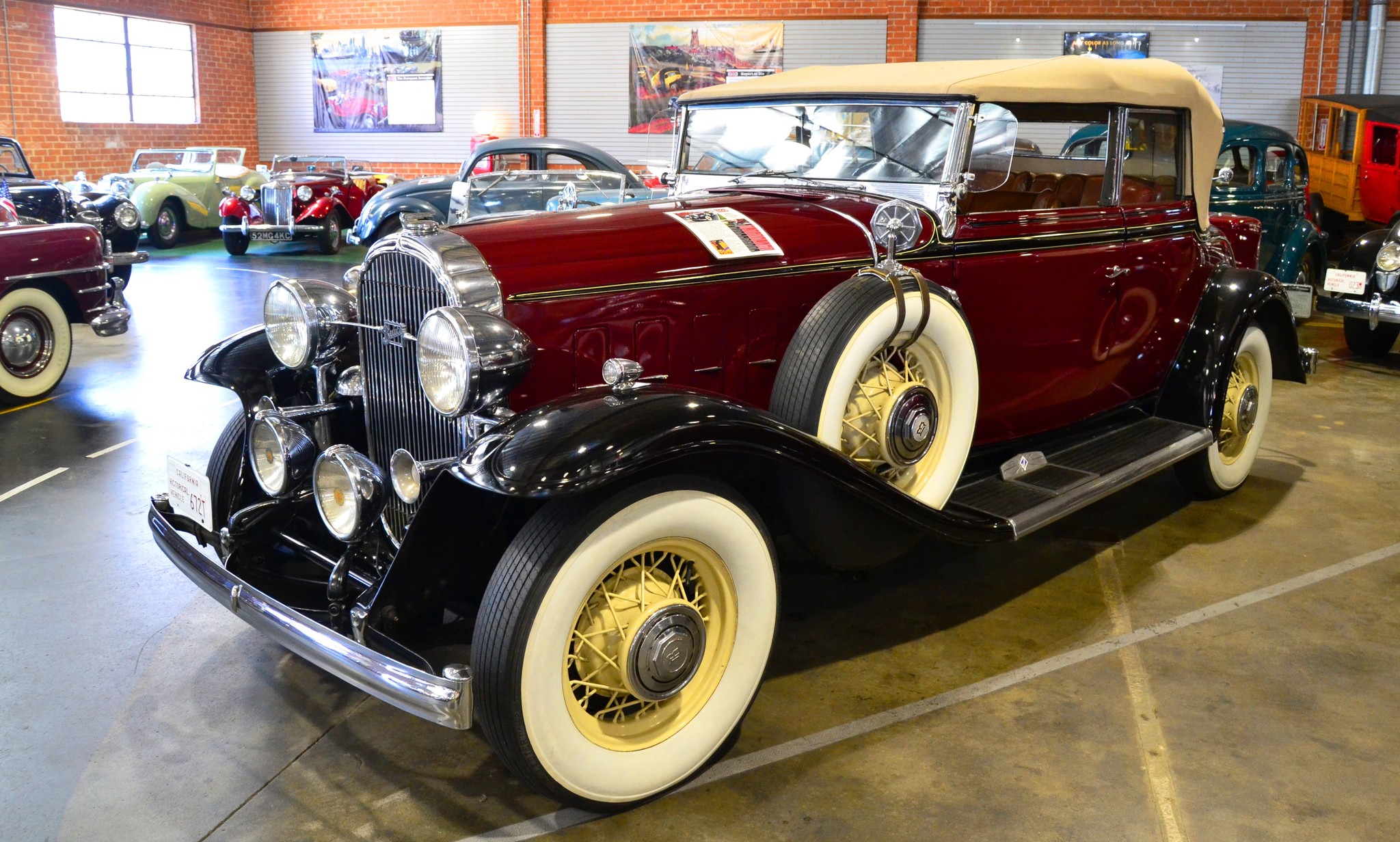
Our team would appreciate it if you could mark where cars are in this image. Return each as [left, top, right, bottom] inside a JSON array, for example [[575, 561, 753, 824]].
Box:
[[0, 131, 863, 414], [1313, 209, 1400, 369], [1058, 115, 1329, 330], [1294, 91, 1400, 258]]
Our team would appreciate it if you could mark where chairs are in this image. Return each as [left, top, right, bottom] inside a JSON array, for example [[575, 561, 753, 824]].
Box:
[[956, 188, 1065, 215]]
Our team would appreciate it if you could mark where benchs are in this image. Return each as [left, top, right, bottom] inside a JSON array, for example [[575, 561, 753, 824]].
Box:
[[1214, 169, 1280, 188], [962, 168, 1164, 207]]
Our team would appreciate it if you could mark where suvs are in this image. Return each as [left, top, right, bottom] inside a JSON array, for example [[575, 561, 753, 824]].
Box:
[[141, 51, 1322, 821]]
